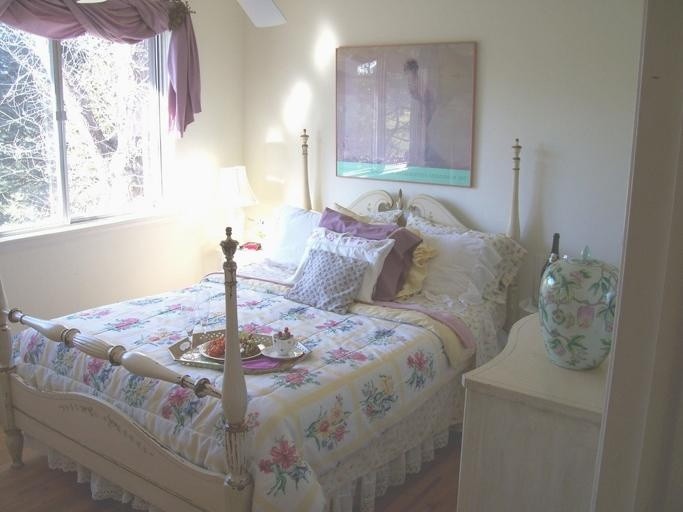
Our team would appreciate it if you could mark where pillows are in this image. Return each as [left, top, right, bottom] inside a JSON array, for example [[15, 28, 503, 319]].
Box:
[[261, 202, 527, 316]]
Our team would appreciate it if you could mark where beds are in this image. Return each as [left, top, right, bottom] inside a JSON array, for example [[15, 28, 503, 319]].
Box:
[[1, 125, 529, 511]]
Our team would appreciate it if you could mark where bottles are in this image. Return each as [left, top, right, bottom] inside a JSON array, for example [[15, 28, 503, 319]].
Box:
[[539, 232, 561, 276]]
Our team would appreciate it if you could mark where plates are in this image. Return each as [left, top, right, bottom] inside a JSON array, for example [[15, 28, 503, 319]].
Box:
[[261, 345, 303, 359], [198, 340, 265, 362]]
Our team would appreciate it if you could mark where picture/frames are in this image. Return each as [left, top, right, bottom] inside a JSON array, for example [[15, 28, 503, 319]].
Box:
[[333, 40, 477, 188]]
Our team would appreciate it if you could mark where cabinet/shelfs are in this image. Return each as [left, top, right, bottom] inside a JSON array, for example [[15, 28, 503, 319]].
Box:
[[456, 310, 612, 510]]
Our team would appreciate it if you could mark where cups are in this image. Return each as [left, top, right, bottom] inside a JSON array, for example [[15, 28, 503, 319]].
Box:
[[271, 332, 298, 357]]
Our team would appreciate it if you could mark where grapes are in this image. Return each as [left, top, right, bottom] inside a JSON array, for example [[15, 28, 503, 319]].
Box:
[[206, 332, 260, 358]]
